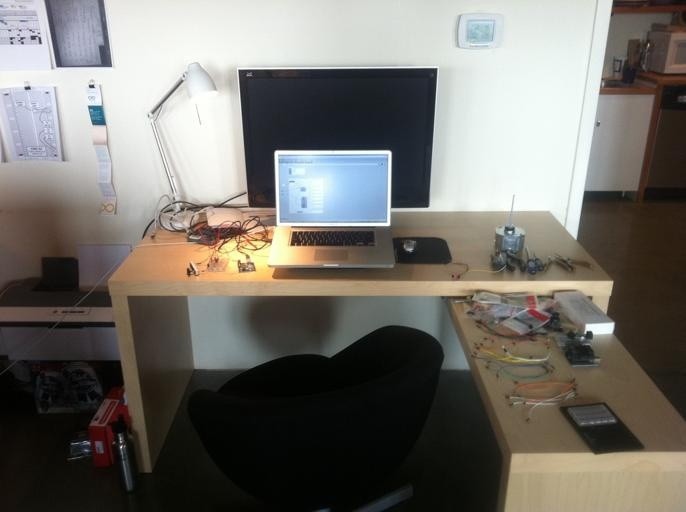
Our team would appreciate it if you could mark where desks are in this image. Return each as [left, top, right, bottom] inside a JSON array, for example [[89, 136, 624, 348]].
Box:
[[108, 211, 686, 512], [636, 71, 686, 203]]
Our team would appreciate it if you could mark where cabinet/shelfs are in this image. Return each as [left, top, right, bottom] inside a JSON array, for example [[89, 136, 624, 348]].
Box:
[[585, 94, 656, 192]]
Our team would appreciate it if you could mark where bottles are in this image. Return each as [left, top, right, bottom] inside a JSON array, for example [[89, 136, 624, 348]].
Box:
[[110, 422, 136, 492]]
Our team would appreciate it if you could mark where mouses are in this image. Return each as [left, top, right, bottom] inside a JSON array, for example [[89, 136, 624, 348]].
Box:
[[400, 239, 417, 255]]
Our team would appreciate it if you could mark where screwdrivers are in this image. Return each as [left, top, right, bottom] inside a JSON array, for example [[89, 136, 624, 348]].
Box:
[[526, 241, 537, 275], [533, 245, 544, 271]]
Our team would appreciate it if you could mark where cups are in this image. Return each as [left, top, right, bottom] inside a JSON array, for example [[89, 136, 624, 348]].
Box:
[[622, 60, 638, 84]]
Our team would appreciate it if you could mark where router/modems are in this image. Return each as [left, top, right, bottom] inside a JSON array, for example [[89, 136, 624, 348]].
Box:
[[207, 207, 245, 229]]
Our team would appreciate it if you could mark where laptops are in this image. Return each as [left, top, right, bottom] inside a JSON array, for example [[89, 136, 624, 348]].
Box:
[[266, 150, 396, 268], [32, 257, 78, 291]]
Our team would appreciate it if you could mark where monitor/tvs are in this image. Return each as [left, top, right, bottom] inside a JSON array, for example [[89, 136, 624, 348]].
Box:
[[238, 68, 438, 208]]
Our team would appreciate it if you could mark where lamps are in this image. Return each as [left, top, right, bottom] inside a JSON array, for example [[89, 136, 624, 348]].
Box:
[[145, 62, 219, 231]]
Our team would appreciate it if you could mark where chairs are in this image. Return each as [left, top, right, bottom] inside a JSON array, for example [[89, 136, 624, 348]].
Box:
[[186, 326, 442, 512]]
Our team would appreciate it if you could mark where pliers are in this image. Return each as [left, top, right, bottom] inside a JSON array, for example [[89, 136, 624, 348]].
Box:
[[505, 250, 525, 273]]
